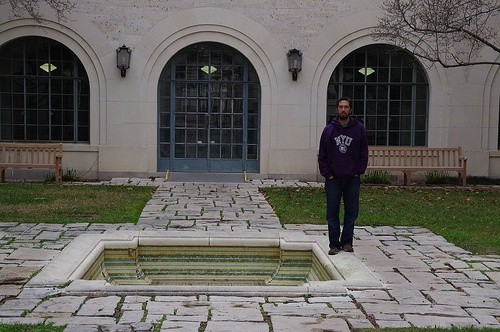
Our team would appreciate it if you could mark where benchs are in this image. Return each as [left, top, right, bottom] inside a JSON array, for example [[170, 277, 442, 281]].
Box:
[[0, 142, 63, 185], [366, 144, 469, 188]]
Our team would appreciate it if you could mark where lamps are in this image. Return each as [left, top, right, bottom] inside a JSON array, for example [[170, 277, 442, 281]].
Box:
[[116, 43, 132, 78], [286, 47, 304, 82]]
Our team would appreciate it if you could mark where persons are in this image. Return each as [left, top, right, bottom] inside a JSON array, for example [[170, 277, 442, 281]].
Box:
[[318, 97, 369, 255]]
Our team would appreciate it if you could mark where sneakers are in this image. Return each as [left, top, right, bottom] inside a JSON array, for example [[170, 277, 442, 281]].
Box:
[[328, 247, 341, 255], [342, 245, 353, 252]]
